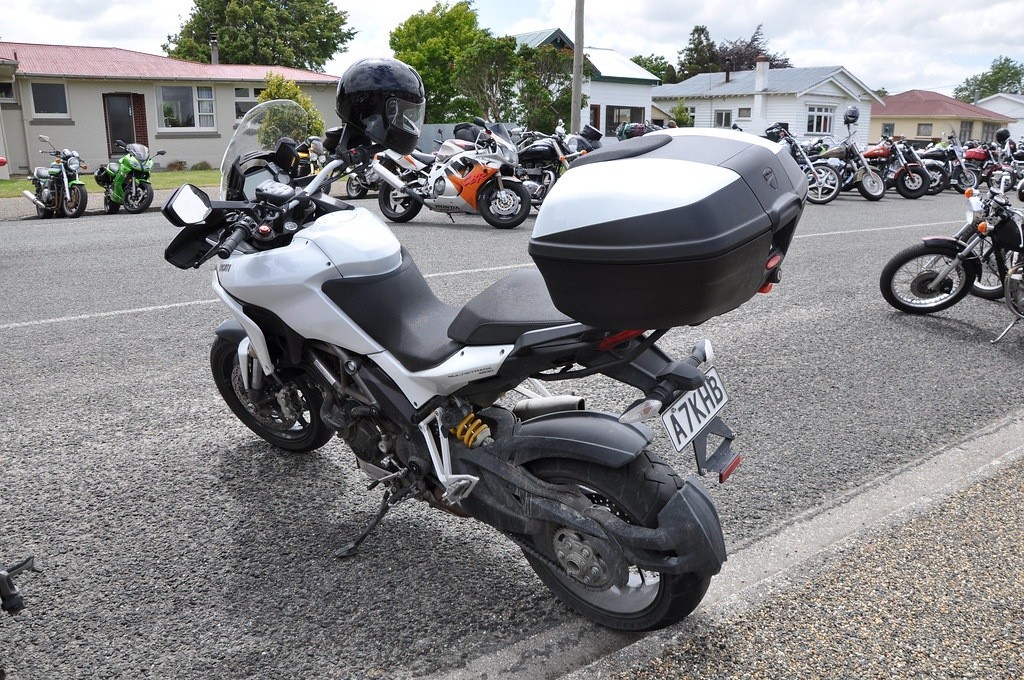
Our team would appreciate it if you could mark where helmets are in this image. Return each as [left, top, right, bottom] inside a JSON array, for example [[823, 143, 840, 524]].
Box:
[[844, 106, 859, 123], [335, 58, 424, 156], [996, 129, 1010, 144]]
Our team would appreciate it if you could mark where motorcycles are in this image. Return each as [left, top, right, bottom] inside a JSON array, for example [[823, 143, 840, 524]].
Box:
[[901, 140, 948, 196], [296, 135, 331, 194], [765, 124, 842, 204], [373, 117, 531, 229], [21, 134, 90, 218], [819, 106, 886, 201], [864, 126, 931, 199], [916, 135, 980, 194], [164, 56, 809, 632], [93, 139, 167, 214], [879, 176, 1024, 346], [518, 120, 587, 211], [965, 143, 1016, 193], [346, 164, 386, 199]]
